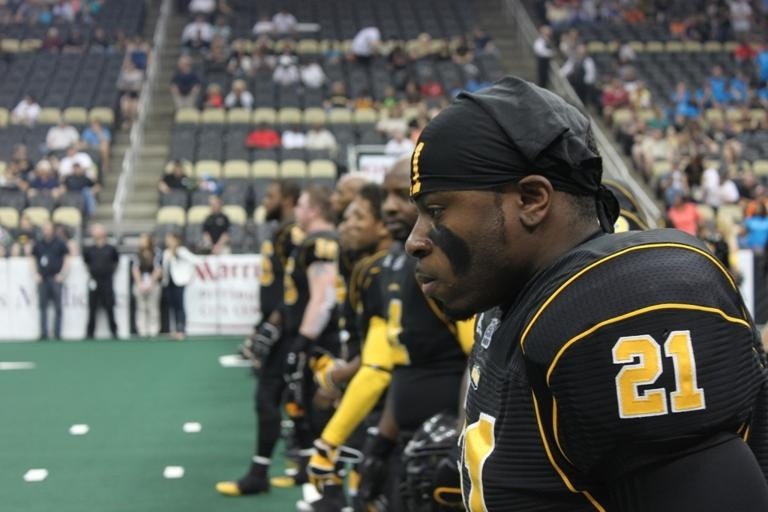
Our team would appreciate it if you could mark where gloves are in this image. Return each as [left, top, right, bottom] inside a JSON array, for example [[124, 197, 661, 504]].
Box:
[[347, 452, 387, 512], [239, 323, 279, 367], [307, 439, 352, 497], [307, 351, 344, 398]]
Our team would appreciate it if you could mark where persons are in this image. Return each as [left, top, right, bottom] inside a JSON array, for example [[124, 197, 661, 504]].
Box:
[[4, 0, 768, 285], [78, 222, 122, 340], [129, 231, 163, 337], [33, 220, 67, 345], [157, 231, 198, 340], [214, 79, 767, 510]]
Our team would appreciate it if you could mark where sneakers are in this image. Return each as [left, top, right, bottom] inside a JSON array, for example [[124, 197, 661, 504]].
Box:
[[269, 466, 305, 487], [295, 489, 350, 512], [214, 470, 272, 497]]
